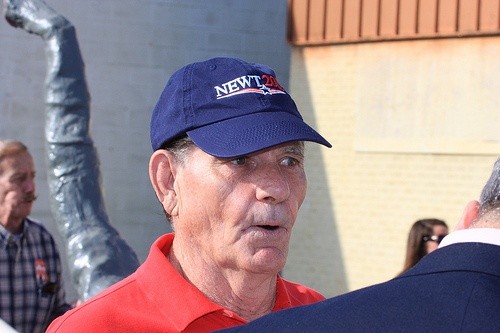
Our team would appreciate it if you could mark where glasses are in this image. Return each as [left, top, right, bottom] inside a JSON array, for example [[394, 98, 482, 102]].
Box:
[[423, 235, 446, 244]]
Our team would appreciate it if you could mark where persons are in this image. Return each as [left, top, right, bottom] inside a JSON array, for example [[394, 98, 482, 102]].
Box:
[[45, 57, 331, 332], [0, 141, 73, 332], [405, 218, 450, 270], [210, 157, 500, 333]]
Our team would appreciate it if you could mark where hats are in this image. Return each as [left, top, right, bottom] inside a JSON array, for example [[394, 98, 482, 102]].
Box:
[[149, 57, 333, 158]]
